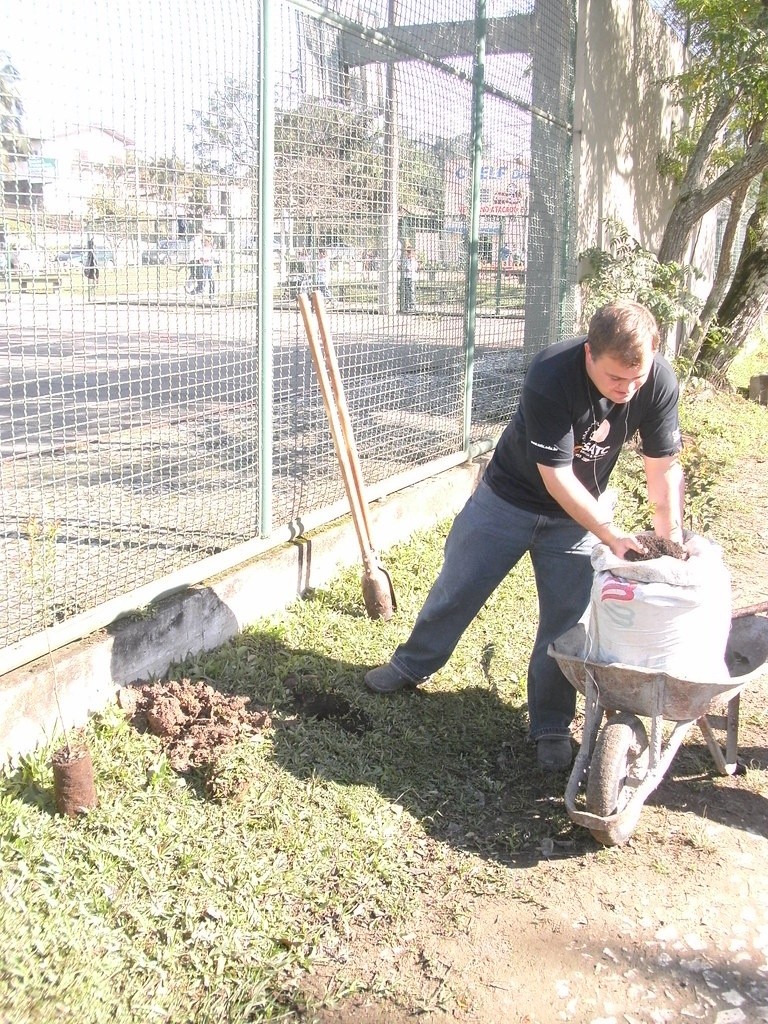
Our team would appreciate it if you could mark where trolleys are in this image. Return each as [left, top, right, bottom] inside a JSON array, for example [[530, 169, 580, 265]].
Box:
[[546, 599, 768, 846]]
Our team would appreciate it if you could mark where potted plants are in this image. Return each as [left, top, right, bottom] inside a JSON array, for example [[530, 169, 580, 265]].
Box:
[[19, 514, 98, 818]]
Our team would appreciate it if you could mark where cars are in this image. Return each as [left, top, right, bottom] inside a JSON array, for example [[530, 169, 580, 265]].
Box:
[[55, 232, 117, 276], [142, 240, 195, 265]]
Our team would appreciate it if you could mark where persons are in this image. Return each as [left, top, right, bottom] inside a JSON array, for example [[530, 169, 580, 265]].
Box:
[[189, 233, 216, 299], [364, 300, 690, 773], [501, 243, 512, 267], [311, 248, 338, 309], [397, 246, 415, 312]]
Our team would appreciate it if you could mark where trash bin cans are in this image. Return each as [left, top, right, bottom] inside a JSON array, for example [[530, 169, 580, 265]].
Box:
[[185, 259, 215, 295]]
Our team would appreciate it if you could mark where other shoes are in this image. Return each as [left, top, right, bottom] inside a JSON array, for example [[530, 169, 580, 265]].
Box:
[[537, 737, 572, 772], [364, 662, 411, 694]]
